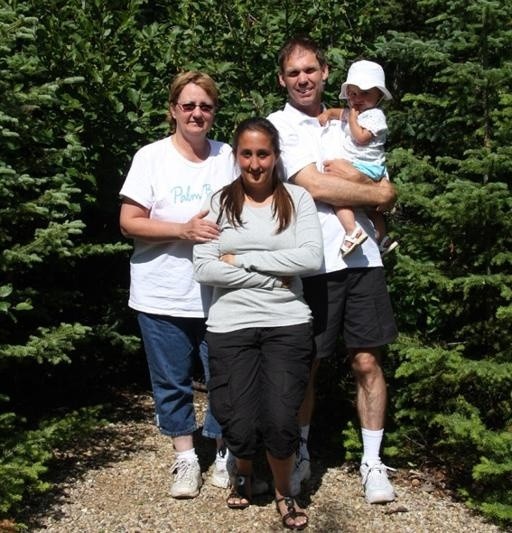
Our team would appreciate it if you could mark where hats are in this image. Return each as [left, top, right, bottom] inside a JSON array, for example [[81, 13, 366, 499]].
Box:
[[338, 59, 393, 101]]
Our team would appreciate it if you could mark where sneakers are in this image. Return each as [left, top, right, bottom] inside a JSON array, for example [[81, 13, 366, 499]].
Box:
[[170, 456, 202, 497], [288, 452, 312, 496], [360, 456, 396, 503], [210, 447, 229, 488]]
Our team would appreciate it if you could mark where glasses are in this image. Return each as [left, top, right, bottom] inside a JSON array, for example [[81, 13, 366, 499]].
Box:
[[178, 103, 214, 113]]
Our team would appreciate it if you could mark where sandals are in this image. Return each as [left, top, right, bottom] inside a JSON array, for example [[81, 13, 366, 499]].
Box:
[[377, 237, 398, 257], [226, 474, 251, 507], [340, 227, 368, 258], [277, 495, 308, 529]]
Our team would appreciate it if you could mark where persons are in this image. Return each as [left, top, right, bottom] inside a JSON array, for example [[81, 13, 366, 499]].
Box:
[[192, 117, 325, 528], [117, 69, 240, 498], [318, 57, 398, 259], [262, 33, 400, 502]]
